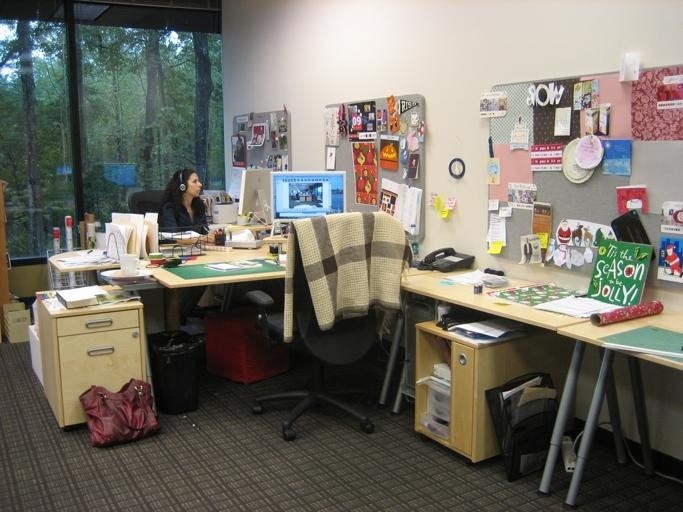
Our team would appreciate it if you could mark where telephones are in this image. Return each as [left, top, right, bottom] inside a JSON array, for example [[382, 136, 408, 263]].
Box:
[[423, 247, 475, 273]]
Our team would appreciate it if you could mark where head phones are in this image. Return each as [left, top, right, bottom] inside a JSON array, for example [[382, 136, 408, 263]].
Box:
[[179, 170, 187, 192]]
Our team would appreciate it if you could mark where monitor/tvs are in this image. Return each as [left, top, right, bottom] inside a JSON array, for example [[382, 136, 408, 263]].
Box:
[[238, 168, 273, 225], [270, 172, 346, 223]]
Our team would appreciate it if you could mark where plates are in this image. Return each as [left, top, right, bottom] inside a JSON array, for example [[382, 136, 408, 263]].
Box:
[[100, 269, 153, 280]]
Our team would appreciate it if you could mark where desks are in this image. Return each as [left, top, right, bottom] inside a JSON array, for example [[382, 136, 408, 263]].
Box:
[[556, 287, 683, 509], [389, 266, 678, 497], [47, 229, 475, 409]]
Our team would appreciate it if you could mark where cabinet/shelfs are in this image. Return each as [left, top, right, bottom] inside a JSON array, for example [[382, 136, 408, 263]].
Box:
[[413, 315, 549, 464], [34, 285, 148, 432]]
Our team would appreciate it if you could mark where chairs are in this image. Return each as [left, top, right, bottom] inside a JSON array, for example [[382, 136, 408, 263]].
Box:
[[243, 211, 412, 442], [125, 189, 170, 228]]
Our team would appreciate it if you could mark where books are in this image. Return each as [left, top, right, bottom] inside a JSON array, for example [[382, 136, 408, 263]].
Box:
[[517, 388, 556, 408], [56, 288, 99, 308], [105, 209, 159, 262]]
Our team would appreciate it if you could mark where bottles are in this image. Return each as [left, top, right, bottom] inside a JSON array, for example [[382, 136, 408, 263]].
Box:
[[474, 281, 483, 294]]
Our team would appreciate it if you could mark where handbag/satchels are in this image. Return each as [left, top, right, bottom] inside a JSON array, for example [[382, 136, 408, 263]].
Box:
[[76, 376, 165, 451]]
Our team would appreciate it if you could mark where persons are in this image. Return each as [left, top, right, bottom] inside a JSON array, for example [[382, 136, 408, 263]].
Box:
[[160, 169, 208, 321]]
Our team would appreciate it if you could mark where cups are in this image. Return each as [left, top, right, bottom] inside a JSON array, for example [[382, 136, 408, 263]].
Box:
[[236, 215, 252, 225], [149, 252, 166, 265], [119, 254, 139, 275]]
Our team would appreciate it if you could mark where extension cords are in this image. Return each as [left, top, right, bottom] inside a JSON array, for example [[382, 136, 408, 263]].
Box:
[[561, 435, 577, 472]]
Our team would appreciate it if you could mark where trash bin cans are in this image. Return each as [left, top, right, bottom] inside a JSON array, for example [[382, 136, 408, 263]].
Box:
[[147, 329, 207, 414]]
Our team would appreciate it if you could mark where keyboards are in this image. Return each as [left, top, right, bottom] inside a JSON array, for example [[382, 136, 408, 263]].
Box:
[[279, 253, 286, 263]]
